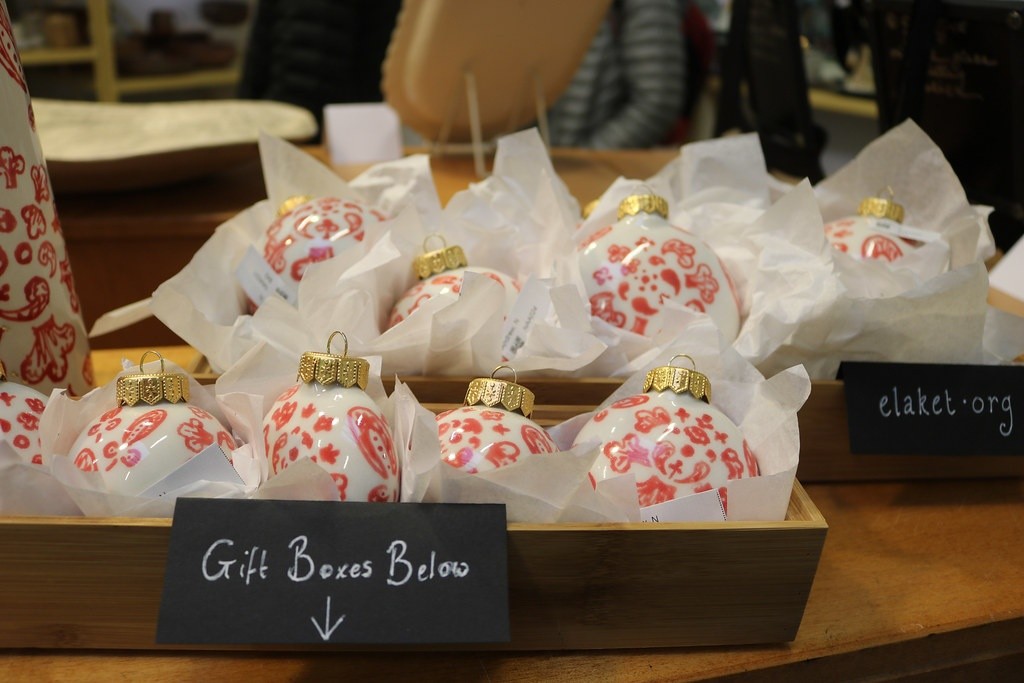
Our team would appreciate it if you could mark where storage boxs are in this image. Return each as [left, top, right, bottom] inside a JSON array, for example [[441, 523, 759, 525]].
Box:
[[0, 403, 832, 649], [192, 286, 1024, 483]]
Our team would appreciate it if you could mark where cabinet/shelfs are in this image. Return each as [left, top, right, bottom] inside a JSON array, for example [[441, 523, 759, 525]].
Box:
[[18, 0, 244, 103]]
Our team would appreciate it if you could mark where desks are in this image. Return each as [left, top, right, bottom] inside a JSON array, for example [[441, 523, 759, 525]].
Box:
[[0, 142, 1024, 683]]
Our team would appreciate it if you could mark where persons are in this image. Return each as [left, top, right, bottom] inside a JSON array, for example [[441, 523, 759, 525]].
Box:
[[681, 0, 1024, 255], [542, 0, 684, 168]]
